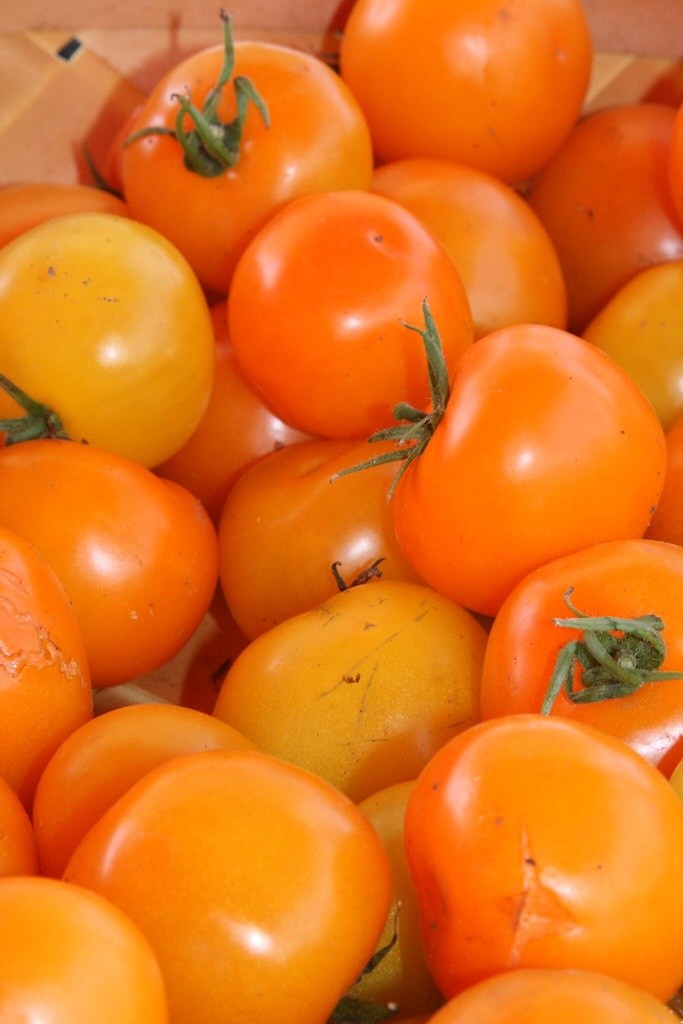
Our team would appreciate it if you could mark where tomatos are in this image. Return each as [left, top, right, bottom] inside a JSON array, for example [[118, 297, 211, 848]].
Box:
[[0, 0, 683, 1024]]
